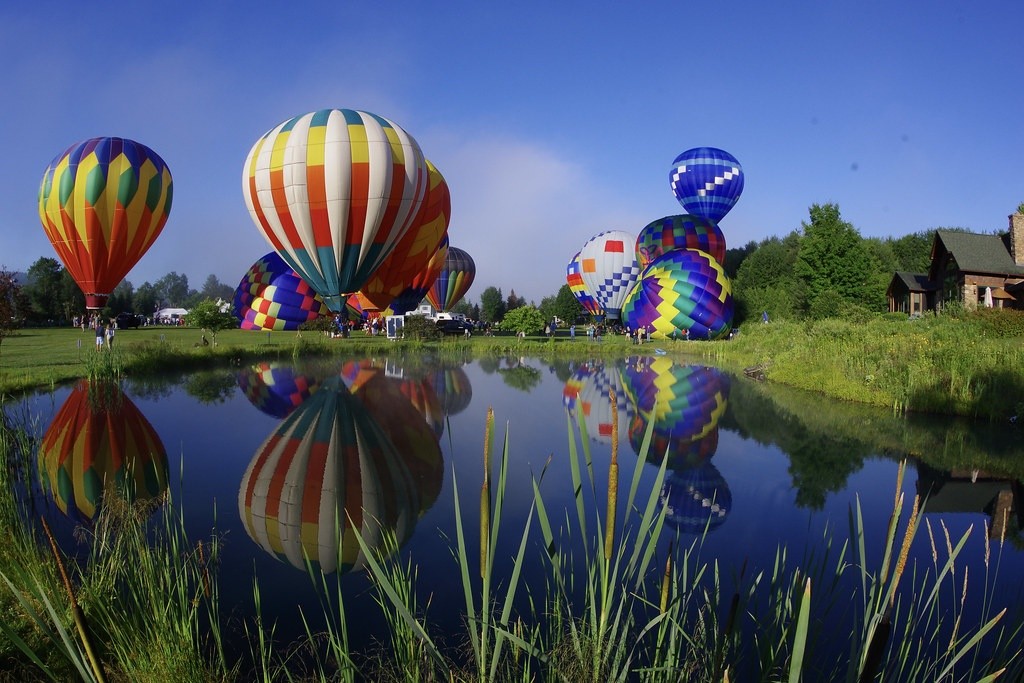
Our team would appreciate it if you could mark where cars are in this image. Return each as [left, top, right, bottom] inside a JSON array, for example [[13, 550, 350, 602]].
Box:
[[115, 313, 141, 329], [436, 320, 474, 337]]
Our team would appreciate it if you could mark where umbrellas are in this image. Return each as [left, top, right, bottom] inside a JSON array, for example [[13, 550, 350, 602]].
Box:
[[981, 287, 1016, 308], [983, 287, 994, 308]]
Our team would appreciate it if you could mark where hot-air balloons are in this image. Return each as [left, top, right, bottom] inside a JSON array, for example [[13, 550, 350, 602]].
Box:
[[38, 137, 174, 330], [563, 352, 733, 535], [37, 376, 170, 532], [228, 109, 476, 339], [233, 358, 474, 578], [565, 146, 745, 342]]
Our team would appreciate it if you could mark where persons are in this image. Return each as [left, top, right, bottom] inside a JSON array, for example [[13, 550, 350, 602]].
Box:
[[544, 322, 557, 338], [763, 310, 770, 324], [296, 325, 302, 338], [471, 321, 492, 336], [622, 355, 651, 376], [332, 313, 385, 338], [584, 321, 652, 345], [73, 312, 179, 333], [570, 325, 576, 341], [96, 323, 105, 353], [673, 327, 690, 341], [708, 329, 712, 341], [105, 324, 114, 350]]
